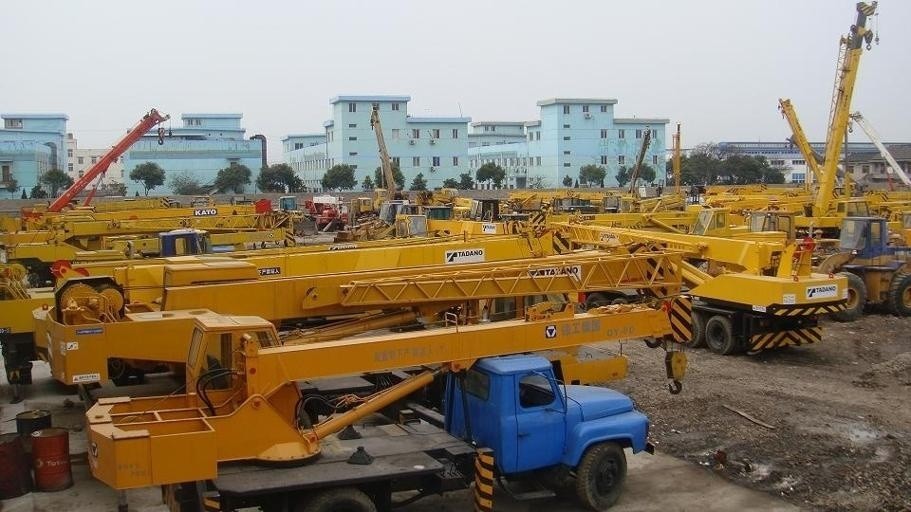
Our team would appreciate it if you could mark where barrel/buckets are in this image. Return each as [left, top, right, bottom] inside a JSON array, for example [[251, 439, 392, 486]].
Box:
[[30, 427, 73, 492], [0, 432, 34, 501], [15, 409, 52, 460]]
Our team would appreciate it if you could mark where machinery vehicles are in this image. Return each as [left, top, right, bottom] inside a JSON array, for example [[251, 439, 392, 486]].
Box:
[[44, 238, 693, 511]]
